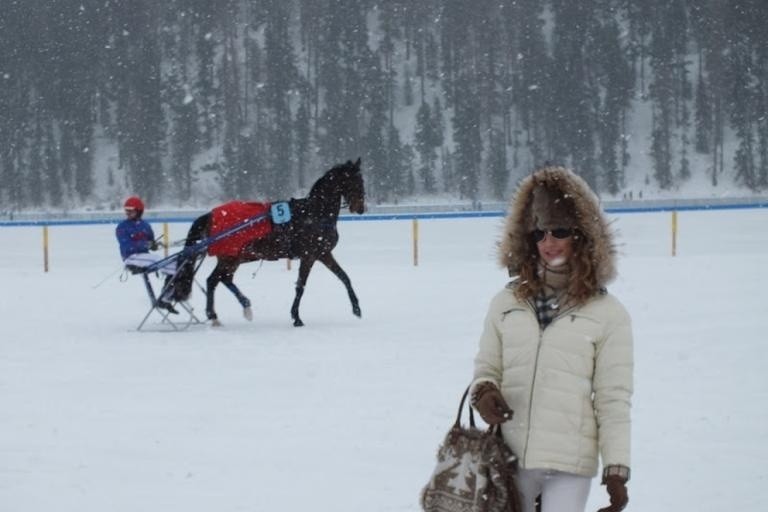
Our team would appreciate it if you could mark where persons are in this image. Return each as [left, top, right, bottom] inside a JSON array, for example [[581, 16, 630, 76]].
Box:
[[116, 196, 180, 314], [468, 165, 635, 512]]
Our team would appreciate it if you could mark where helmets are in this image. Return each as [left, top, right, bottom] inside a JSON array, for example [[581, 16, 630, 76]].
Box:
[[124, 198, 143, 218]]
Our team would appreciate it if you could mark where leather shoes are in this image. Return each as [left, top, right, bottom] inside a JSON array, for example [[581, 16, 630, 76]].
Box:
[[158, 301, 178, 313]]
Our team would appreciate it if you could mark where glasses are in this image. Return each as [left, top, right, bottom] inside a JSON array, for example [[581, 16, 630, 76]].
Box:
[[531, 229, 571, 244]]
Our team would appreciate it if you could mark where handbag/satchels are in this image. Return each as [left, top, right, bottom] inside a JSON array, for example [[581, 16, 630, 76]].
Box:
[[422, 376, 521, 512]]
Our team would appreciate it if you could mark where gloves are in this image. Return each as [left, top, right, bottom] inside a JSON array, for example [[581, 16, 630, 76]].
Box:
[[597, 475, 628, 512], [473, 382, 513, 424]]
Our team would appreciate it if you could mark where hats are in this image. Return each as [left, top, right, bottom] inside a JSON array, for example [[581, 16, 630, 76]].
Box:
[[524, 186, 578, 236]]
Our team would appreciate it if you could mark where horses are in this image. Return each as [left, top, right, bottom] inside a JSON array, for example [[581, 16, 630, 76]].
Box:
[[174, 157, 366, 327]]
